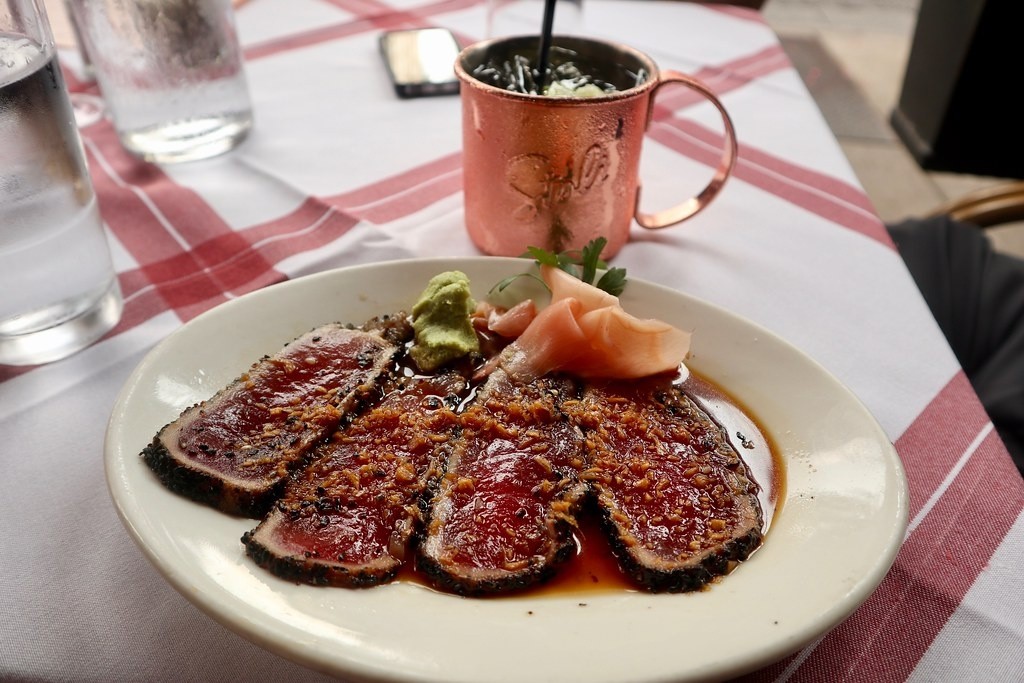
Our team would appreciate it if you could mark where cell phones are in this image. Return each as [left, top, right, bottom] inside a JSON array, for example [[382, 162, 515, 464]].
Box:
[[380, 27, 462, 97]]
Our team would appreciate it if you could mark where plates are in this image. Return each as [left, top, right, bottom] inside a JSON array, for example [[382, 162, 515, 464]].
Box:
[[104, 256, 911, 683]]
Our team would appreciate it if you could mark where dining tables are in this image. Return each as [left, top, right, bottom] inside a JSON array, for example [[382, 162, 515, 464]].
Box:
[[0, 0, 1024, 683]]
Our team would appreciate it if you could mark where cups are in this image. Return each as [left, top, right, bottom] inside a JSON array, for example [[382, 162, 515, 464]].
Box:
[[1, 0, 126, 366], [67, 0, 254, 163], [455, 31, 737, 264], [38, 0, 107, 128]]
